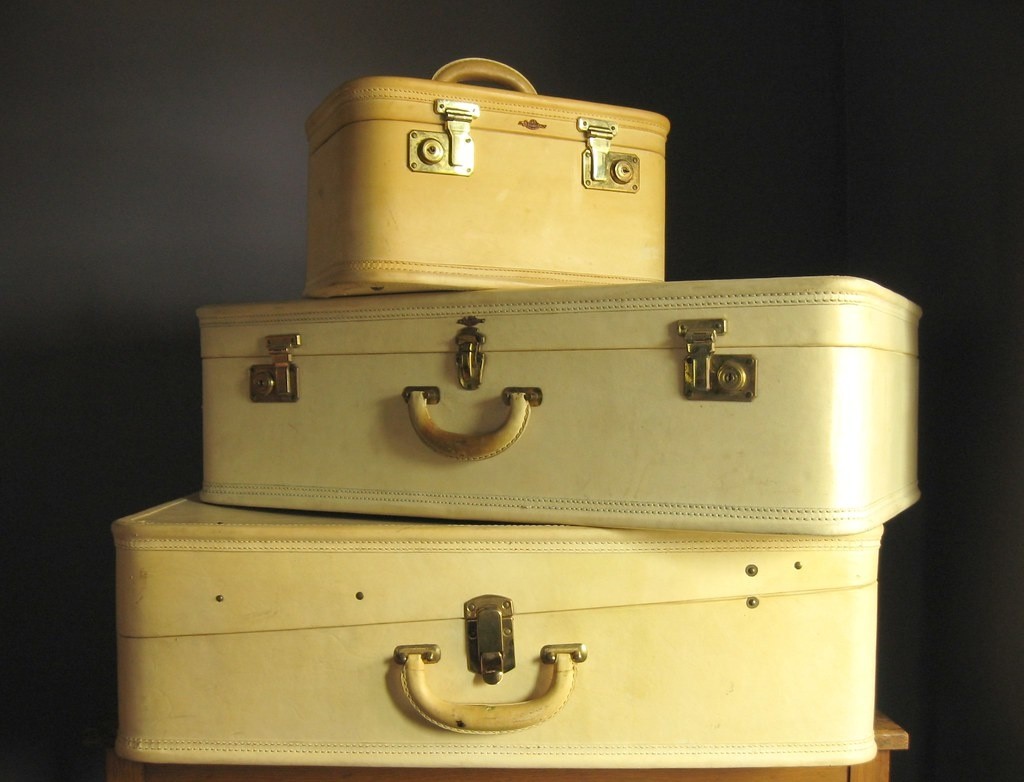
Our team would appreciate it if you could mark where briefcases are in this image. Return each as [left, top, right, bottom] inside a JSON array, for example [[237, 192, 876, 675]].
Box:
[[110, 500, 885, 769], [196, 273, 921, 538], [301, 58, 669, 298]]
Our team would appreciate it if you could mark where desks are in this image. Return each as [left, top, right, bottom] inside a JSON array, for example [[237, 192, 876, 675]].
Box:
[[106, 709, 908, 782]]
